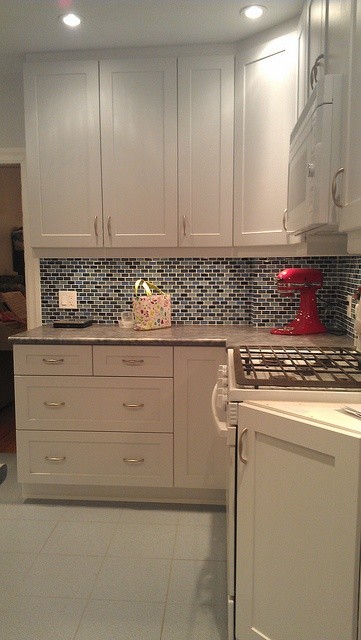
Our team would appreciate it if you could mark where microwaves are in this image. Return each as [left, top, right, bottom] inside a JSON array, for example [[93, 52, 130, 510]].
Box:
[[280, 73, 349, 238]]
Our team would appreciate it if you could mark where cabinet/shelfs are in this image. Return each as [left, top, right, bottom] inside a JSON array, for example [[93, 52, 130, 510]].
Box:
[[229, 402, 360, 640], [178, 53, 234, 248], [297, 0, 327, 116], [22, 54, 178, 248], [235, 32, 299, 247], [326, 2, 360, 231], [14, 345, 227, 505]]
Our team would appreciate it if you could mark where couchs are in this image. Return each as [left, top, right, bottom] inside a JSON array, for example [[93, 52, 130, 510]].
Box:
[[0, 274, 26, 354]]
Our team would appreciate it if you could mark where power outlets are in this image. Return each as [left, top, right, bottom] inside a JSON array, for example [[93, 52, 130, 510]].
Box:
[[347, 296, 351, 318], [59, 290, 77, 309]]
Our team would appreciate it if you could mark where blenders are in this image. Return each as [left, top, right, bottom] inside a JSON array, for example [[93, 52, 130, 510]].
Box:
[[268, 270, 328, 336]]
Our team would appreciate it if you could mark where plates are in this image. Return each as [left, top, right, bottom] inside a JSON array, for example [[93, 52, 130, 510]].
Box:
[[117, 320, 134, 328]]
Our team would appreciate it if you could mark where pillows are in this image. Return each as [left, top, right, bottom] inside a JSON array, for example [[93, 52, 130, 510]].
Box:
[[3, 291, 26, 319]]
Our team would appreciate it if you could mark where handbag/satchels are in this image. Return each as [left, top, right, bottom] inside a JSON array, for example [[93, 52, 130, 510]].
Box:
[[133, 280, 171, 330]]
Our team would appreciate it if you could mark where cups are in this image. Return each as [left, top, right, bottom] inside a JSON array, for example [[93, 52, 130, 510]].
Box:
[[122, 311, 132, 326]]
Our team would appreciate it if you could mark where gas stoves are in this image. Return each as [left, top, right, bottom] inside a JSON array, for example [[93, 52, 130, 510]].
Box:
[[210, 347, 361, 424]]
